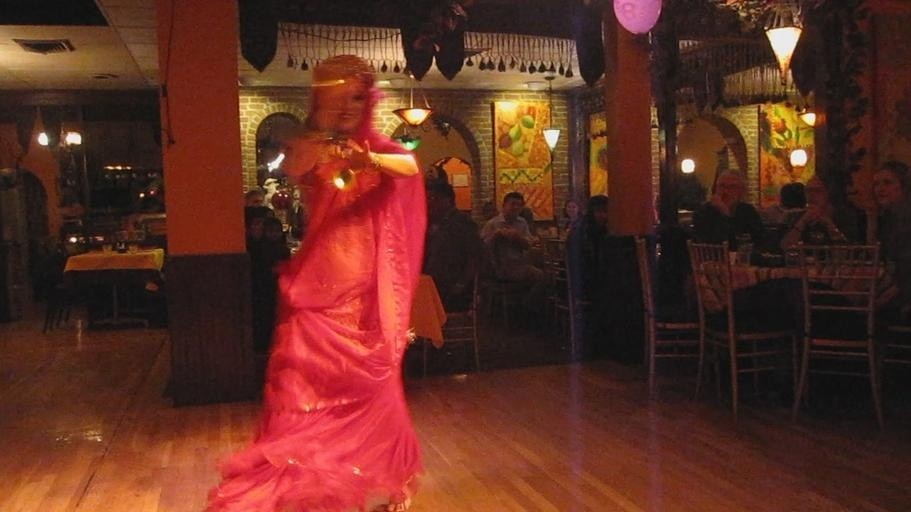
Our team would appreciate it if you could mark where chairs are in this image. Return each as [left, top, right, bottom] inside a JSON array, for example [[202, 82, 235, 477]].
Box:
[[39, 237, 94, 333]]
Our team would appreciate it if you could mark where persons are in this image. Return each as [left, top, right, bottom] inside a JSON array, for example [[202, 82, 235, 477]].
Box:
[[205, 54, 427, 512], [243, 187, 300, 353], [408, 162, 911, 391]]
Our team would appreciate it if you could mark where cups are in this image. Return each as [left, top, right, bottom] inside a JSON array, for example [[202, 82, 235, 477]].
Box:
[[100, 239, 139, 254], [734, 231, 753, 269]]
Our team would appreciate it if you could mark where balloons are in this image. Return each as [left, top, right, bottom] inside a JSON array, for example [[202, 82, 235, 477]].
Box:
[[613, 0, 662, 34]]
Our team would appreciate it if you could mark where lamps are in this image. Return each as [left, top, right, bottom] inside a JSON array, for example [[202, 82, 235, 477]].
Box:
[[393, 74, 432, 127], [543, 72, 561, 152], [764, 0, 802, 83]]
